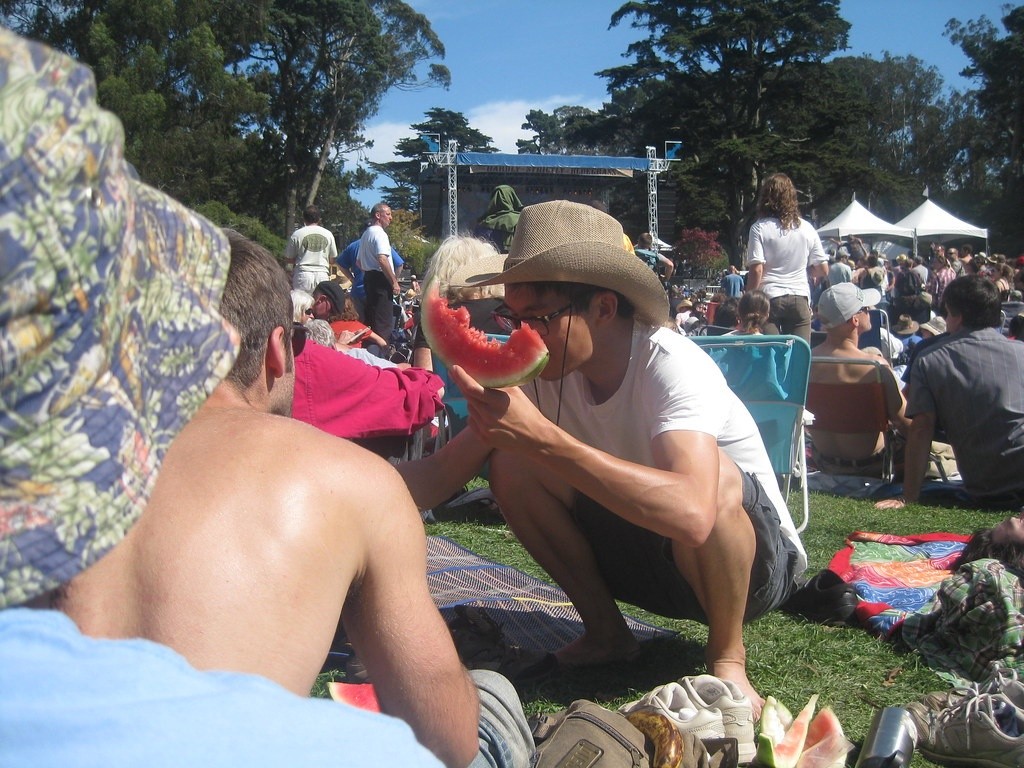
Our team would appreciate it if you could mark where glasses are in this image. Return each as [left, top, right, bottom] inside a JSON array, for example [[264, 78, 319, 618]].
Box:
[[305, 308, 314, 316], [490, 300, 575, 336], [290, 322, 308, 358], [327, 297, 337, 316], [948, 252, 955, 255], [843, 256, 847, 259], [856, 307, 867, 315]]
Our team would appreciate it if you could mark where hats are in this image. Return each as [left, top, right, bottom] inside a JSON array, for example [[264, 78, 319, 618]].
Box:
[[818, 283, 881, 328], [892, 314, 919, 334], [879, 254, 888, 262], [896, 254, 908, 264], [450, 200, 670, 328], [676, 299, 692, 312], [987, 254, 998, 264], [836, 249, 850, 259], [0, 26, 240, 612], [317, 281, 346, 314], [920, 316, 946, 336], [975, 252, 987, 260]]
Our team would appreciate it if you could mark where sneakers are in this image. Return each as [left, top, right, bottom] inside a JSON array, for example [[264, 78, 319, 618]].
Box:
[[903, 660, 1024, 768], [617, 674, 757, 764]]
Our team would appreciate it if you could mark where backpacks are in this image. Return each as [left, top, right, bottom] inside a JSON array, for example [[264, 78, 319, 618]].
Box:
[[528, 699, 739, 768]]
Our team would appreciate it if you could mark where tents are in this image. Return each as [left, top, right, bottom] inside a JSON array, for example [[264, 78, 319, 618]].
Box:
[[816, 198, 988, 258], [633, 231, 673, 251]]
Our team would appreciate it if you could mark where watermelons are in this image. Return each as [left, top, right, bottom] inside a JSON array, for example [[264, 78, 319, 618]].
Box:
[[421, 275, 549, 387], [325, 681, 379, 711]]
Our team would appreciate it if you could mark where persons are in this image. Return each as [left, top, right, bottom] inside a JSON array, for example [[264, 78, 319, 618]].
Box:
[[634, 232, 674, 282], [809, 234, 1023, 391], [588, 200, 635, 255], [955, 512, 1024, 578], [745, 173, 829, 345], [0, 29, 536, 768], [805, 282, 913, 477], [393, 200, 809, 722], [873, 274, 1024, 512], [665, 261, 770, 336]]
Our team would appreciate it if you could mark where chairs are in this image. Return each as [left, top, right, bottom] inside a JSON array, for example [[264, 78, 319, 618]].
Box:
[[685, 307, 954, 532], [429, 331, 511, 482]]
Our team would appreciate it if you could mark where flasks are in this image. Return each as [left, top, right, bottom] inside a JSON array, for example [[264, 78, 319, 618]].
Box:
[[855, 704, 919, 768]]
